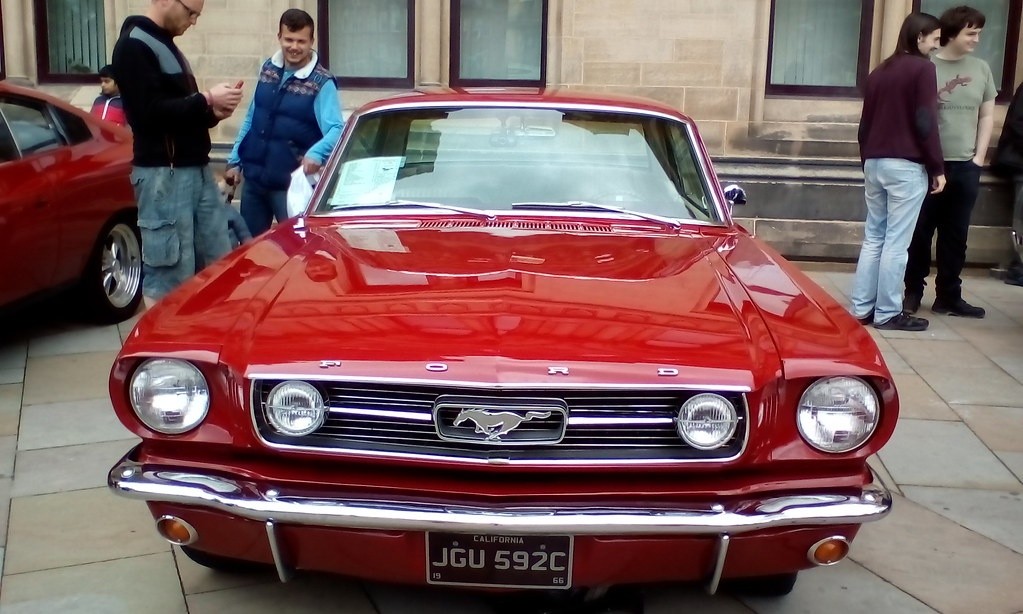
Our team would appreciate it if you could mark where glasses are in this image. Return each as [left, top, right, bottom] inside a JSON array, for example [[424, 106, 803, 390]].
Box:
[[176, 0, 200, 17]]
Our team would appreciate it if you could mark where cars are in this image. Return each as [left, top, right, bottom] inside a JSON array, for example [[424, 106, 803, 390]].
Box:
[[0, 76, 143, 324], [108, 87, 900, 609]]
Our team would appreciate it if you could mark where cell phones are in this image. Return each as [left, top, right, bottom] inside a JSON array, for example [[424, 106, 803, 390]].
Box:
[[234, 80, 243, 89]]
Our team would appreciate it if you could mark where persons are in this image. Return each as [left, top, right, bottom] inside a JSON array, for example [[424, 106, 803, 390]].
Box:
[[112, 1, 244, 307], [211, 171, 253, 250], [851, 11, 947, 330], [88, 62, 128, 130], [223, 8, 346, 242], [901, 6, 998, 320]]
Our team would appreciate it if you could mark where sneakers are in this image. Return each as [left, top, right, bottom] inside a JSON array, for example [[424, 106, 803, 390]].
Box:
[[1005, 263, 1023, 286], [902, 292, 923, 313], [931, 285, 986, 319], [858, 310, 929, 331]]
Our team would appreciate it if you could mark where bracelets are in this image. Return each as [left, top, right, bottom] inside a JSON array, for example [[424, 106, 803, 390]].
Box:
[[207, 90, 215, 107]]
